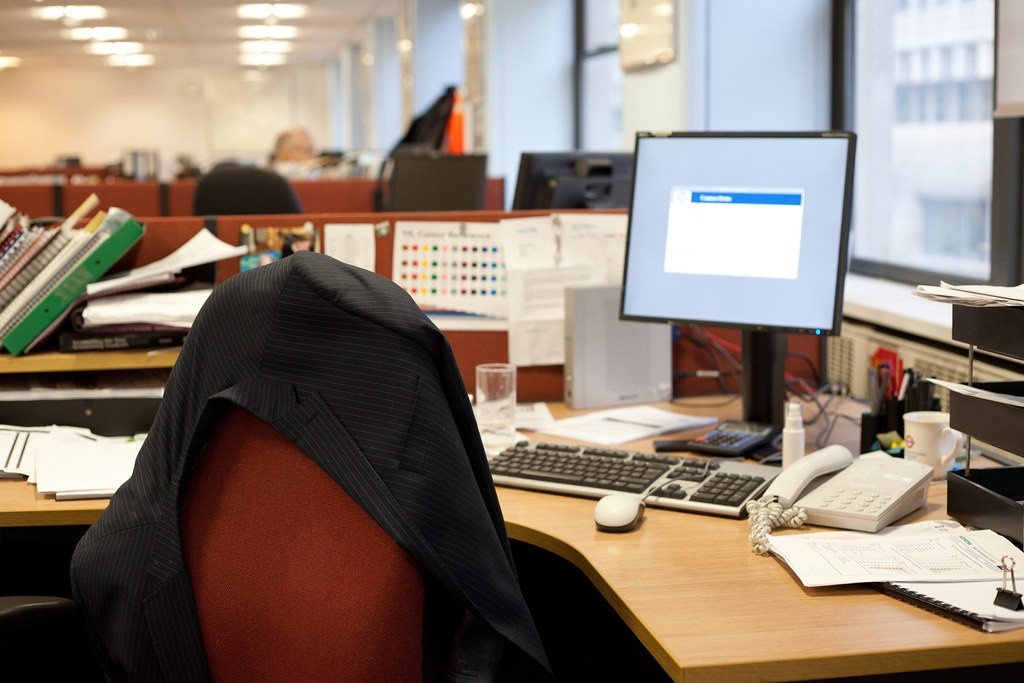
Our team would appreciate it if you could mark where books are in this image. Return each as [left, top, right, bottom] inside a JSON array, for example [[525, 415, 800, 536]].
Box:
[[870, 529, 1024, 633], [0, 193, 213, 357]]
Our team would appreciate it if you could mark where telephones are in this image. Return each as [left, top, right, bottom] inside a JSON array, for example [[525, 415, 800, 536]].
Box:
[[746, 445, 934, 555]]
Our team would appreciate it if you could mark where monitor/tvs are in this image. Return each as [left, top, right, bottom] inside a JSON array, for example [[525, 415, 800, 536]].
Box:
[[388, 151, 486, 212], [512, 153, 635, 211], [619, 131, 858, 463]]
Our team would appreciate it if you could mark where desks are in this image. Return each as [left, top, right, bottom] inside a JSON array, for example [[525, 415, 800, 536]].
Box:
[[0, 378, 1024, 683]]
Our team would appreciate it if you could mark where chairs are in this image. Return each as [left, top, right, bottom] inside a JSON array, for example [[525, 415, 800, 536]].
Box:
[[153, 256, 424, 683]]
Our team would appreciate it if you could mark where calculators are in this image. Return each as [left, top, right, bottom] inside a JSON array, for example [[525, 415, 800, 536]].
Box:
[[687, 419, 772, 455]]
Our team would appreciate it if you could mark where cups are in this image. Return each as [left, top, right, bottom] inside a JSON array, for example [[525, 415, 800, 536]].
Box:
[[903, 411, 962, 481], [475, 363, 516, 439]]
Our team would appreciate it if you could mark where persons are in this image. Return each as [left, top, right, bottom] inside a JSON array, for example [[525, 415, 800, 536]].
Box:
[[277, 128, 315, 159]]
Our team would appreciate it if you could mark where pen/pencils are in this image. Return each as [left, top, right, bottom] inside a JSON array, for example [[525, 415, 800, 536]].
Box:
[[898, 367, 913, 403]]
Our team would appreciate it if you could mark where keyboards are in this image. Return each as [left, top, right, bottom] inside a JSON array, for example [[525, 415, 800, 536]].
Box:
[[488, 441, 783, 517]]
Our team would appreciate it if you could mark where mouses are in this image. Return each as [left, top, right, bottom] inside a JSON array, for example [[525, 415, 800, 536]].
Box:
[[594, 494, 646, 532]]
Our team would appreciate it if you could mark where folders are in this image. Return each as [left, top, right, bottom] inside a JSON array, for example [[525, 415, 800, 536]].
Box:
[[3, 215, 144, 355]]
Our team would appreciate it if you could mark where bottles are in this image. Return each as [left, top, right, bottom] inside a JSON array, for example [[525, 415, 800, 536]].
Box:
[[782, 402, 805, 467]]
